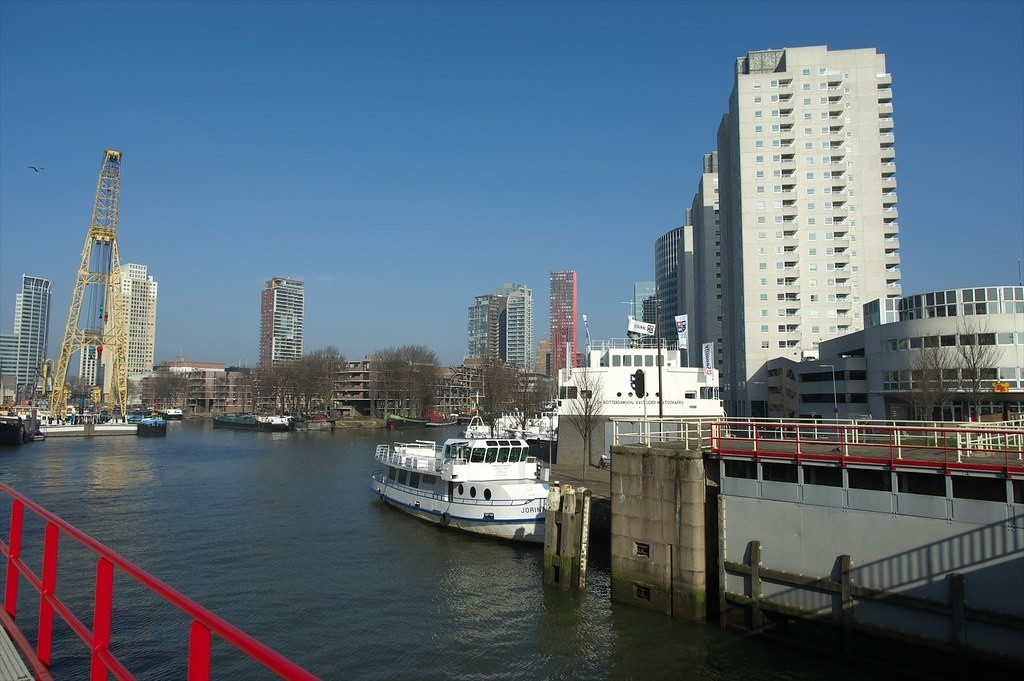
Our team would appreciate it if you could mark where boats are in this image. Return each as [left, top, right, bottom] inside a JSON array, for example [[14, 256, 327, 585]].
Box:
[[212, 411, 295, 431], [370, 438, 563, 542], [462, 301, 736, 464], [156, 406, 182, 420], [426, 406, 459, 426], [386, 413, 426, 430]]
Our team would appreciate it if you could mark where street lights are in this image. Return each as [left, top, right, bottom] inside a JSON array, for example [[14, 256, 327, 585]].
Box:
[[819, 365, 840, 437]]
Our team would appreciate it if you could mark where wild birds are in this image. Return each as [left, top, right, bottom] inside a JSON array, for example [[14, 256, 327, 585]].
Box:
[[27, 166, 45, 173]]
